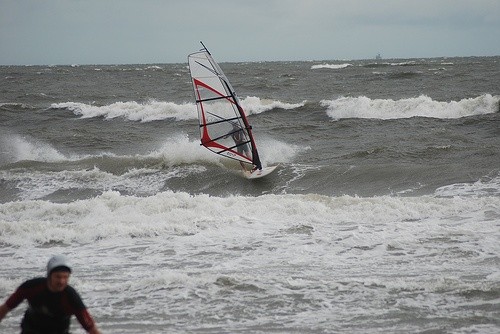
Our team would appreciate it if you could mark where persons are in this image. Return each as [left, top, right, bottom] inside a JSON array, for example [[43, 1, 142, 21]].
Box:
[[0, 252, 104, 334], [225, 119, 256, 171]]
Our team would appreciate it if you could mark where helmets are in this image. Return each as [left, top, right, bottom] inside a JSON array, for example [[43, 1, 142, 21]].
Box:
[[47, 255, 72, 277]]
